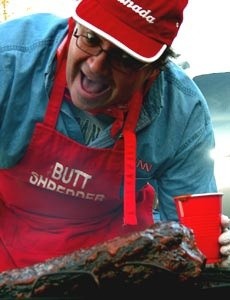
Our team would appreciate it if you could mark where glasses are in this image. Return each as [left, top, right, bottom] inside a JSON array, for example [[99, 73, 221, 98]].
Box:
[[73, 27, 148, 75]]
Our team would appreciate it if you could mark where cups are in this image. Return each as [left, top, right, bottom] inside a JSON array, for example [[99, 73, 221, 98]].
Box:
[[173, 191, 224, 264]]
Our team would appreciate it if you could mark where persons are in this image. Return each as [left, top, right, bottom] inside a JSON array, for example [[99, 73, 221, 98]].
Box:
[[0, 0, 230, 269]]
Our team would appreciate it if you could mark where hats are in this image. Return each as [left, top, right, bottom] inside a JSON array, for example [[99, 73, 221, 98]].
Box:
[[73, 0, 189, 64]]
